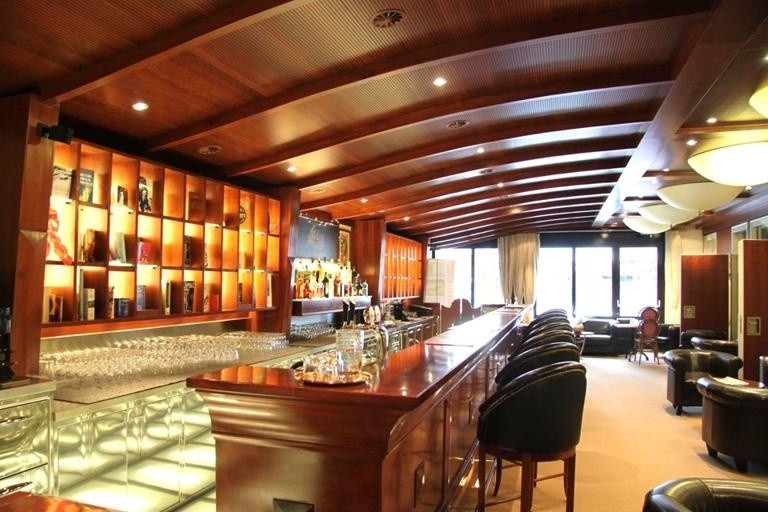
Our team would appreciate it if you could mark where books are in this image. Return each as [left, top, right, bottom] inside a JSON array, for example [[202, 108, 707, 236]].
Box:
[[184, 281, 196, 314], [108, 284, 147, 319], [76, 268, 84, 323], [84, 288, 95, 321], [42, 287, 65, 323]]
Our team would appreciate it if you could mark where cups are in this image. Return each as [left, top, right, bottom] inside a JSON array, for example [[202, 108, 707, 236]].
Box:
[[302, 328, 364, 382], [38, 330, 287, 396]]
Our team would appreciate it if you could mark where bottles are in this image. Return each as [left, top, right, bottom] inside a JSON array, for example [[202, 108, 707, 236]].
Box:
[[292, 260, 369, 299]]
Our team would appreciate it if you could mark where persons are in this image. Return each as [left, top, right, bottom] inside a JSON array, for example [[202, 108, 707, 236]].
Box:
[[138, 187, 152, 213]]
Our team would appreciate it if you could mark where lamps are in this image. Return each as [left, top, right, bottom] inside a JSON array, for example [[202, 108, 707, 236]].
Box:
[[639, 201, 700, 226], [688, 141, 768, 187], [657, 182, 744, 211], [749, 78, 768, 118], [623, 216, 671, 235]]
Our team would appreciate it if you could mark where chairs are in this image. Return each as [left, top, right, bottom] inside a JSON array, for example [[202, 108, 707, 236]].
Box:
[[630, 308, 661, 364], [663, 350, 768, 472], [479, 308, 587, 511], [640, 477, 768, 512]]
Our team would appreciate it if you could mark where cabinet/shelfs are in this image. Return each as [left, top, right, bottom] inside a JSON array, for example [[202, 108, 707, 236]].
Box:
[[382, 232, 426, 300], [40, 137, 281, 338]]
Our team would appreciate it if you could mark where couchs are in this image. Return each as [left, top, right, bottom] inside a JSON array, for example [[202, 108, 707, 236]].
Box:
[[680, 327, 740, 356], [578, 310, 680, 351]]
